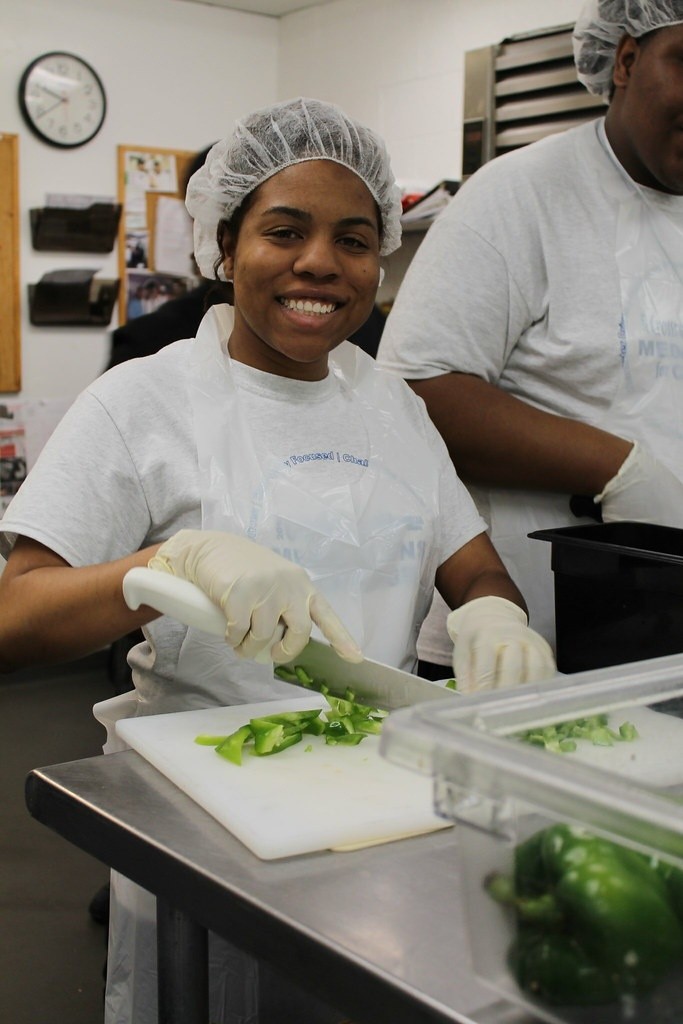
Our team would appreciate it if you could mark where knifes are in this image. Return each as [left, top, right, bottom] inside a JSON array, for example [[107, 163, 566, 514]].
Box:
[[122, 567, 462, 710]]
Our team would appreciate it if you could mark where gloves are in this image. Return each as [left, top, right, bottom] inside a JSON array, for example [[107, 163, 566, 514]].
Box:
[[152, 531, 364, 668], [445, 597, 556, 694], [596, 440, 682, 529]]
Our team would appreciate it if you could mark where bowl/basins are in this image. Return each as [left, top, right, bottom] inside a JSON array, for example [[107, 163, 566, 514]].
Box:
[[526, 521, 683, 674], [380, 653, 682, 1024]]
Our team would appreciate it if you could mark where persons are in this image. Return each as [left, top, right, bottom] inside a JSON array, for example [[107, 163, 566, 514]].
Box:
[[371, 0, 683, 682], [0, 93, 558, 1024]]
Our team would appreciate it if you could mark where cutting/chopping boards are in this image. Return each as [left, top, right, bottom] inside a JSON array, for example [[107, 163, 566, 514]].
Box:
[[114, 693, 454, 860]]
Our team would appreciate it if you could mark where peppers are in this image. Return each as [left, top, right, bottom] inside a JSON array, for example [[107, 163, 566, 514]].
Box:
[[194, 664, 640, 766], [479, 823, 683, 1024]]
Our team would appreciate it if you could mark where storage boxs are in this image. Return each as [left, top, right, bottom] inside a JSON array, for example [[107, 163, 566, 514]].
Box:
[[373, 654, 683, 1024], [525, 518, 683, 673]]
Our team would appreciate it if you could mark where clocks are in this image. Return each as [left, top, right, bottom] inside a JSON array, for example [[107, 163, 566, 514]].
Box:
[[20, 50, 106, 149]]
